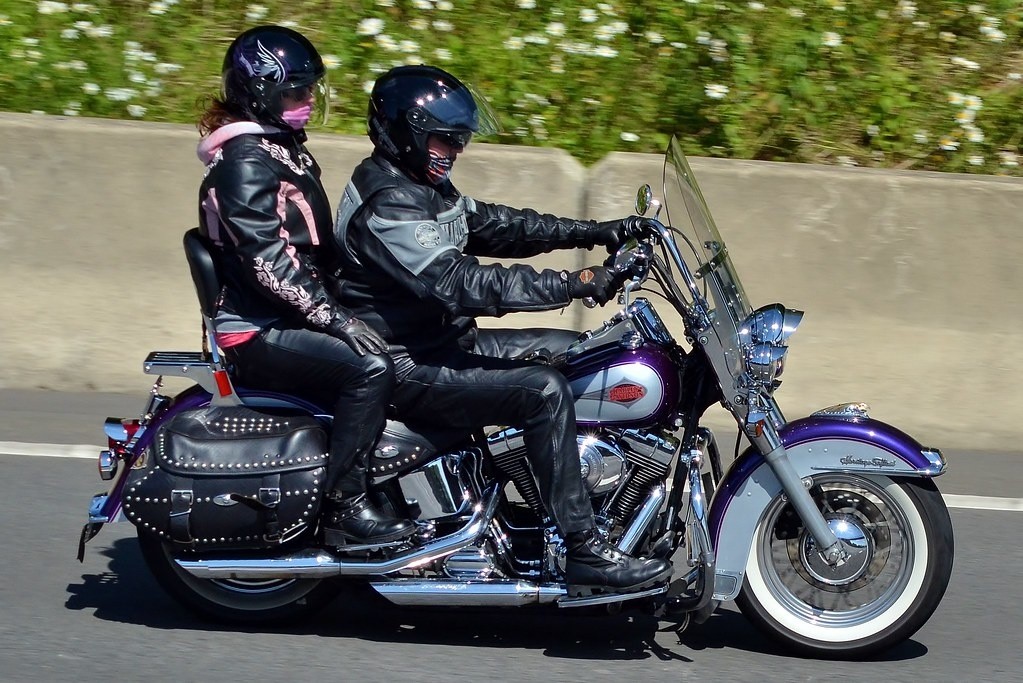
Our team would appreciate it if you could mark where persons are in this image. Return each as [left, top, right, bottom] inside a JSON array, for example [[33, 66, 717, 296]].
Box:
[[197, 25, 415, 542], [337, 65, 675, 600]]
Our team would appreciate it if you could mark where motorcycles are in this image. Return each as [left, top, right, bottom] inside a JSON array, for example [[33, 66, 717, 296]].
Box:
[[71, 135, 957, 657]]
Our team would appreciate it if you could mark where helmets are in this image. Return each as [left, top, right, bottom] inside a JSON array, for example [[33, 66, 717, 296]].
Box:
[[364, 62, 503, 190], [218, 23, 329, 136]]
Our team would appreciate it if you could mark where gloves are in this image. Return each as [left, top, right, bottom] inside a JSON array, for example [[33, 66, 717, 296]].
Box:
[[567, 266, 615, 306], [598, 215, 640, 246], [329, 306, 391, 354]]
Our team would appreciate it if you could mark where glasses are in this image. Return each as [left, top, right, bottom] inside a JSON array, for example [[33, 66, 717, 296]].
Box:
[[280, 78, 317, 100], [435, 129, 469, 147]]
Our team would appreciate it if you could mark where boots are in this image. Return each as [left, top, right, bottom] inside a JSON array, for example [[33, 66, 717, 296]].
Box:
[[316, 461, 413, 547], [564, 526, 673, 596]]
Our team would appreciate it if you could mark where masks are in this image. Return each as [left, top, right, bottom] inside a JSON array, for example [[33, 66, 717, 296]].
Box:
[[276, 105, 310, 130], [426, 148, 456, 187]]
